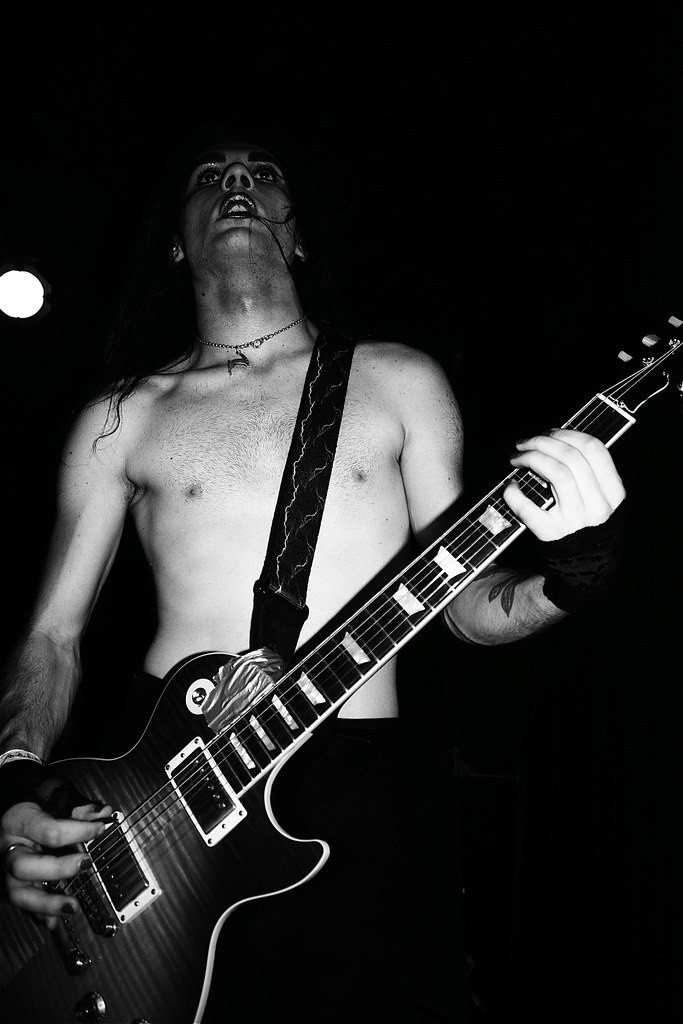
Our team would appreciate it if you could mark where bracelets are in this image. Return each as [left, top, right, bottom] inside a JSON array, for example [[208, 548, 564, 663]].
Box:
[[1, 748, 41, 768]]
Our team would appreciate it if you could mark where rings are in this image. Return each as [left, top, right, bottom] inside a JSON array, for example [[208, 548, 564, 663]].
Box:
[[5, 842, 25, 863]]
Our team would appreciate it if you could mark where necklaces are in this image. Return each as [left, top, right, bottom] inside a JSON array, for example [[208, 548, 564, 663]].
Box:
[[193, 314, 309, 377]]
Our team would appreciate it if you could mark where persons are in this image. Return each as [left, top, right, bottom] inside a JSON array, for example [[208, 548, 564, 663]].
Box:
[[0, 142, 629, 1024]]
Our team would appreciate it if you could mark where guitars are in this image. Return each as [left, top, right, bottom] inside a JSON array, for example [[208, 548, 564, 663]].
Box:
[[0, 317, 683, 1023]]
